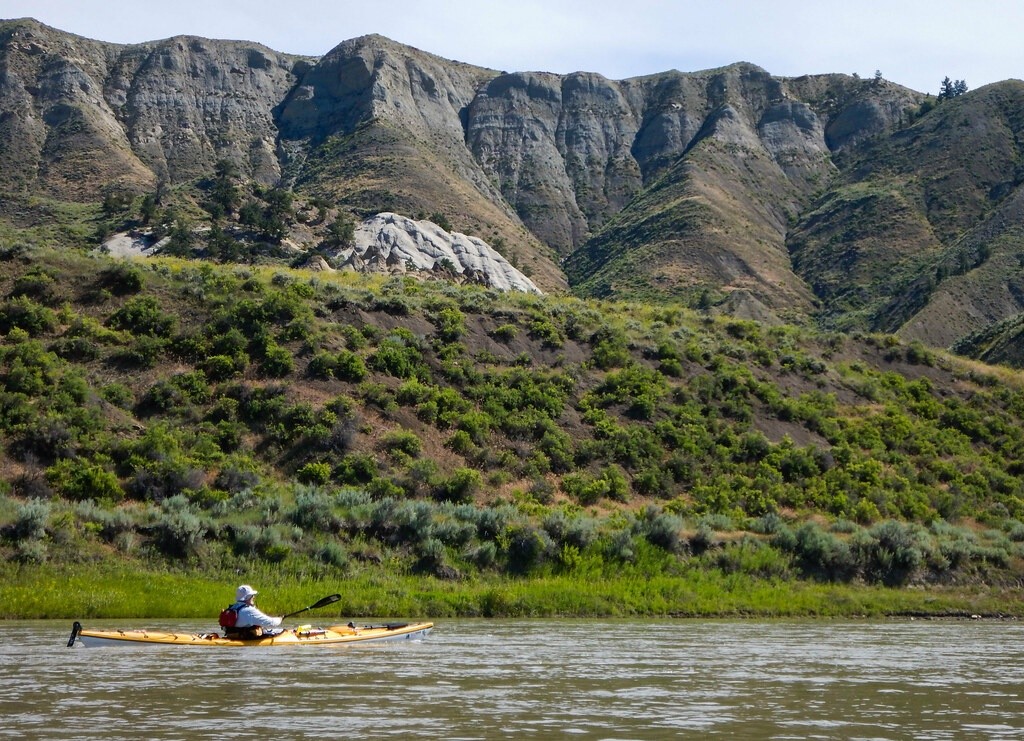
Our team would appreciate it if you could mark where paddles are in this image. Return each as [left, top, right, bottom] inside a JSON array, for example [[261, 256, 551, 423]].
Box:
[[282, 593, 342, 620]]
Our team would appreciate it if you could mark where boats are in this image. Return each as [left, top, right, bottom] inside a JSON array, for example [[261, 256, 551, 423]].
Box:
[[65, 616, 437, 648]]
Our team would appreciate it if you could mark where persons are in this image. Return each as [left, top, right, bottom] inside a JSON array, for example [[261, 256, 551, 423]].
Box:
[[226, 584, 285, 640]]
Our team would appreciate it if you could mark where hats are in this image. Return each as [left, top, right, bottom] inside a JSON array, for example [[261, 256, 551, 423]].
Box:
[[236, 585, 257, 601]]
